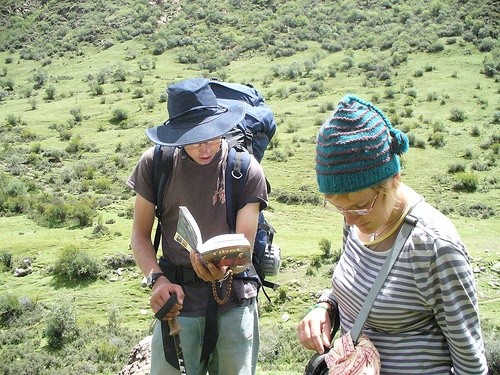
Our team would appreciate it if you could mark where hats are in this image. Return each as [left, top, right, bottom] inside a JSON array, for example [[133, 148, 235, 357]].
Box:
[[313, 95, 411, 195], [142, 76, 247, 147]]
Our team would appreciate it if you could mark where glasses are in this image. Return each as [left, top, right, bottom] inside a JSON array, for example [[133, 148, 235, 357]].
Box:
[[321, 187, 379, 216], [181, 136, 224, 151]]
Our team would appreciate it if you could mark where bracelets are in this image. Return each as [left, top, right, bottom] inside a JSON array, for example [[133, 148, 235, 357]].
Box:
[[307, 304, 331, 321], [212, 272, 232, 305], [317, 299, 335, 313]]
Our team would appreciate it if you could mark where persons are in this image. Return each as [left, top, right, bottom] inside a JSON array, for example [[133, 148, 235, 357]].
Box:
[[125, 78, 269, 375], [297, 94, 489, 375]]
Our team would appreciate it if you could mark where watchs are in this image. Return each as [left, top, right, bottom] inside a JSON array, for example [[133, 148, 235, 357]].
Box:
[[147, 272, 165, 288]]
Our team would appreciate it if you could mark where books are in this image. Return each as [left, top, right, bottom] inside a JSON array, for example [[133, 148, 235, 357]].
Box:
[[173, 206, 252, 269]]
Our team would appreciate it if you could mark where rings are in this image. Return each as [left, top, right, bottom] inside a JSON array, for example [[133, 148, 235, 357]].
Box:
[[300, 331, 304, 334]]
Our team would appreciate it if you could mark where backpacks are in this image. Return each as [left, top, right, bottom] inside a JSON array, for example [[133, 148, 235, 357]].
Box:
[[206, 79, 282, 282]]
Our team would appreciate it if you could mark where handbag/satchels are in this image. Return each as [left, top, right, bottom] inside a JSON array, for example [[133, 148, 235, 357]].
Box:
[[301, 340, 340, 375]]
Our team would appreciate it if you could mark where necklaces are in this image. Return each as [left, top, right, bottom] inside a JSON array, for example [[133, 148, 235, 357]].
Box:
[[369, 203, 407, 242]]
